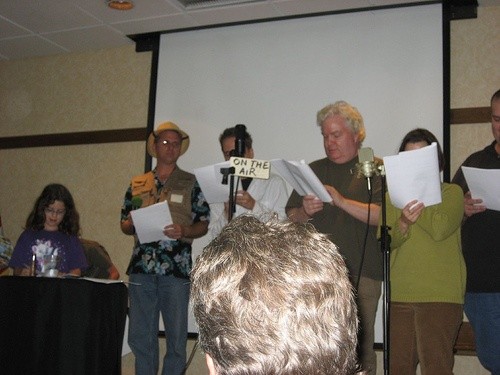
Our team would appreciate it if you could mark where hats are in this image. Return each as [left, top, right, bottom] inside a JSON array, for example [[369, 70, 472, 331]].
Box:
[[147, 122, 190, 158]]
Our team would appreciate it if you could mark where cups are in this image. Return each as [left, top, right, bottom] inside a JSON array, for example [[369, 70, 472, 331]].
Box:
[[43, 254, 60, 278]]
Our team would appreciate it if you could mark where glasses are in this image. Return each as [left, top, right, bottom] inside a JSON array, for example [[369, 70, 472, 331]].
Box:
[[44, 207, 65, 216], [155, 140, 181, 147]]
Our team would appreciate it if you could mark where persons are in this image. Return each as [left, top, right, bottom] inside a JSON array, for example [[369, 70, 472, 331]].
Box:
[[0, 88, 386, 375], [189, 213, 362, 375], [450, 89, 500, 375], [377, 128, 467, 375], [7, 183, 88, 277]]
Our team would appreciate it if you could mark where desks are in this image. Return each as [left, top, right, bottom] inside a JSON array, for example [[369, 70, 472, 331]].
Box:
[[0, 275, 128, 375]]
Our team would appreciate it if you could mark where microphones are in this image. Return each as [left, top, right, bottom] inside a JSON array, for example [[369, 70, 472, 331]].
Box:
[[131, 196, 142, 234], [235, 124, 246, 158], [358, 147, 374, 196]]
